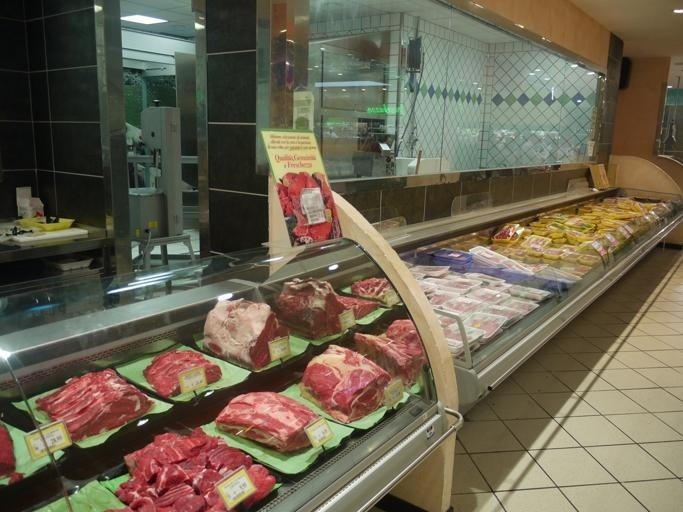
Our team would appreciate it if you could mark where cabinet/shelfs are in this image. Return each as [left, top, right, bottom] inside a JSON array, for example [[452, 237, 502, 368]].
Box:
[[235, 187, 683, 418], [1, 280, 465, 512]]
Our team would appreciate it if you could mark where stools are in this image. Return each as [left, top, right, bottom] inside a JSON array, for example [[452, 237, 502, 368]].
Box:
[[131, 235, 196, 270]]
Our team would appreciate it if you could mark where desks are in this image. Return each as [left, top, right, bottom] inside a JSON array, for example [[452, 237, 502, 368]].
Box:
[[0, 219, 111, 275]]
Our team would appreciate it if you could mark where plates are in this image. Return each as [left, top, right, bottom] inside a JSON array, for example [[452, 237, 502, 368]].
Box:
[[41, 253, 94, 271], [16, 216, 76, 231]]
